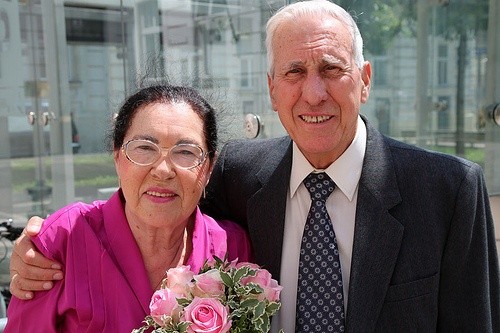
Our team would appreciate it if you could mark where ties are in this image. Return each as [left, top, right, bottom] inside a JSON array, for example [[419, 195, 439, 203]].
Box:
[[296, 172, 345, 333]]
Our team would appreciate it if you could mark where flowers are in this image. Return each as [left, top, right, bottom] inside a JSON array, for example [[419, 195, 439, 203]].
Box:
[[130, 252, 285, 333]]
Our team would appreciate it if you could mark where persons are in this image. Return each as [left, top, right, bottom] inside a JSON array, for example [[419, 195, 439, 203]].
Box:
[[9, 0, 500, 333], [3, 85, 250, 333]]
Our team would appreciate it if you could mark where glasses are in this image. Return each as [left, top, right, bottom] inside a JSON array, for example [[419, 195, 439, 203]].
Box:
[[122, 138, 205, 171]]
